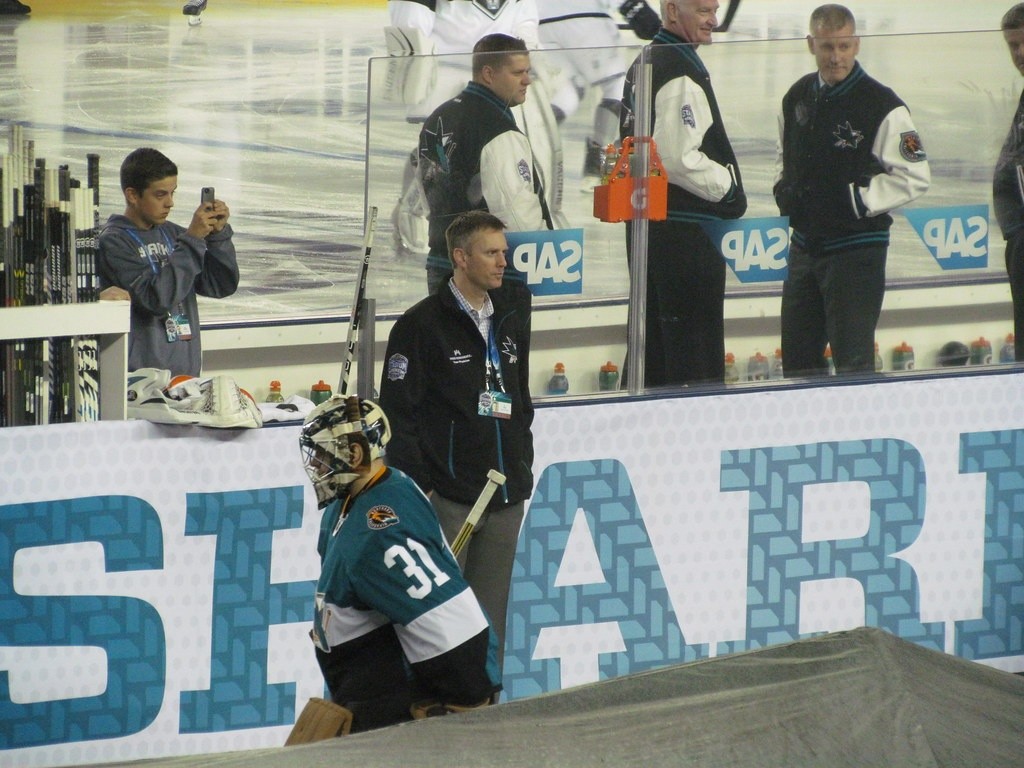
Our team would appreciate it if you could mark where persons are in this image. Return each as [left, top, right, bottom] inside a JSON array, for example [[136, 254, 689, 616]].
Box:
[[99, 147, 239, 377], [620, 0, 747, 389], [383, 0, 563, 258], [418, 33, 554, 385], [309, 394, 494, 733], [379, 209, 535, 701], [993, 4, 1024, 363], [773, 5, 930, 378], [534, 0, 627, 178]]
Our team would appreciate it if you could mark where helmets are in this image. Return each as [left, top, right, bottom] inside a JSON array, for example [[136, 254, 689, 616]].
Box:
[[299, 394, 392, 511]]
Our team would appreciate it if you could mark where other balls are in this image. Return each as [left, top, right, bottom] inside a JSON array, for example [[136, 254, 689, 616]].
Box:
[[276, 403, 299, 412]]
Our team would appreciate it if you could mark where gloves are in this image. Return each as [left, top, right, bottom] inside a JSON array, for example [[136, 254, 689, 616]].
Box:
[[620, 0, 663, 39]]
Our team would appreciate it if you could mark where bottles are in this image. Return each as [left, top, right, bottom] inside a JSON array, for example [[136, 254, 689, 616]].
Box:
[[999, 334, 1016, 363], [598, 361, 620, 393], [824, 345, 837, 376], [547, 362, 569, 395], [310, 380, 332, 408], [873, 342, 883, 374], [264, 380, 284, 403], [724, 352, 739, 383], [772, 348, 783, 379], [747, 352, 770, 382], [892, 342, 914, 371], [970, 336, 993, 367]]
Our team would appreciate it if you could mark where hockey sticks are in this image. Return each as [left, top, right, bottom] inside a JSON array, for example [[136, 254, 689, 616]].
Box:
[[0, 125, 101, 425], [450, 467, 508, 550], [338, 205, 379, 395]]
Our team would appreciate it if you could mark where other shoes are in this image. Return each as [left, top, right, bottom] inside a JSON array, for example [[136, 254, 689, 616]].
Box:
[[184, 0, 208, 15], [580, 139, 606, 177], [0, 0, 31, 14]]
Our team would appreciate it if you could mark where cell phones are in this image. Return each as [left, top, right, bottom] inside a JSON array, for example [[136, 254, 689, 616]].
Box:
[[202, 188, 215, 211]]
